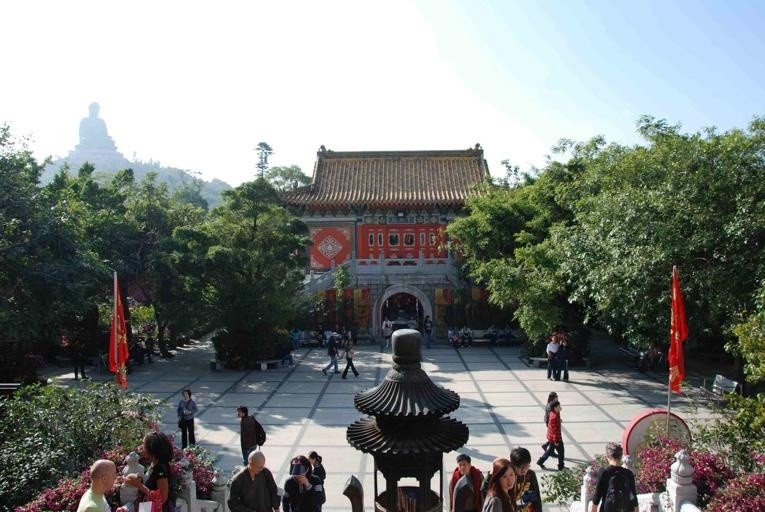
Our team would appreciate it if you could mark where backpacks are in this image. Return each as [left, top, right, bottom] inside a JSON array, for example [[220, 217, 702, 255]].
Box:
[[604, 464, 634, 512]]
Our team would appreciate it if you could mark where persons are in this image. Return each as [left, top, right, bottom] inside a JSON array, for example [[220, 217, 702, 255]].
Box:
[[449, 445, 542, 512], [228, 450, 281, 512], [585, 440, 639, 512], [125, 333, 160, 366], [282, 314, 573, 382], [124, 433, 177, 512], [236, 406, 266, 464], [78, 459, 116, 512], [282, 451, 326, 512], [646, 341, 660, 372], [537, 401, 568, 472], [177, 388, 198, 449], [73, 341, 89, 379], [542, 393, 559, 451]]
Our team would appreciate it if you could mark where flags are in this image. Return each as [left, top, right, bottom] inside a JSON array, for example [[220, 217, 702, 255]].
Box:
[[667, 270, 689, 393], [109, 282, 130, 391]]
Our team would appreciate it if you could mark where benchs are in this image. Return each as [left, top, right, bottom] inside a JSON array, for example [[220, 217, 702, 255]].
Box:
[[303, 331, 353, 345], [699, 374, 743, 410], [448, 330, 516, 346], [208, 359, 227, 369], [256, 359, 282, 371], [615, 342, 648, 361], [529, 357, 568, 368]]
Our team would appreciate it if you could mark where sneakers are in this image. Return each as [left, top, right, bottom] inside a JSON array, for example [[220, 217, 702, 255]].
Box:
[[536, 443, 569, 471], [322, 368, 359, 379], [288, 364, 295, 368]]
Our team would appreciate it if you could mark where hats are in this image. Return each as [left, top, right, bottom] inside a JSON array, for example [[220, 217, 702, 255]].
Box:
[[331, 332, 339, 337], [290, 459, 308, 475]]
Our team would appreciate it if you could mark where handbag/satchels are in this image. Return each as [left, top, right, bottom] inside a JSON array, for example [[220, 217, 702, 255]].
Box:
[[251, 415, 265, 445], [138, 491, 152, 512], [178, 418, 182, 428], [347, 352, 353, 359]]
